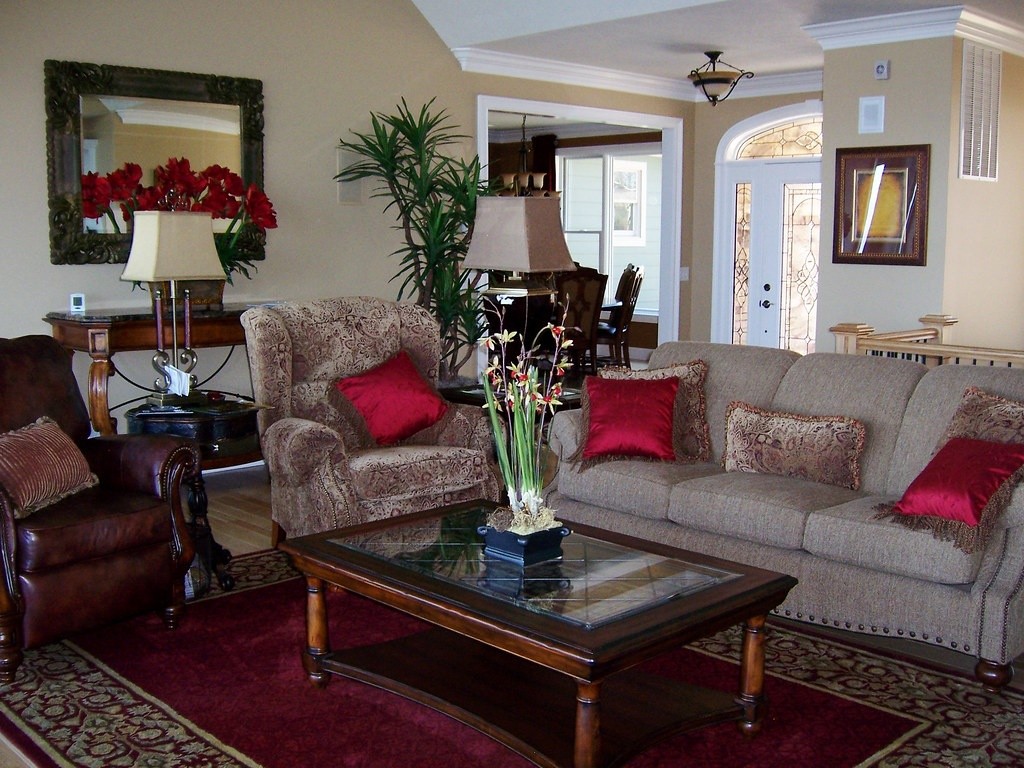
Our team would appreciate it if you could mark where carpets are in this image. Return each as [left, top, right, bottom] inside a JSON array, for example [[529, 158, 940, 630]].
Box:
[[0, 544, 1024, 768]]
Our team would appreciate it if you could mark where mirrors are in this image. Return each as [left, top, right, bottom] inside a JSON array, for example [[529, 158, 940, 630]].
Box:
[[43, 57, 266, 264]]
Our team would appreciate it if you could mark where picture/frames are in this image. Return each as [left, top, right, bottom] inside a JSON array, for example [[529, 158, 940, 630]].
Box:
[[833, 143, 931, 266]]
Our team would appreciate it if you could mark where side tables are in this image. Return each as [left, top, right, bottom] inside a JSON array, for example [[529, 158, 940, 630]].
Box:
[[124, 401, 266, 466], [440, 374, 581, 420]]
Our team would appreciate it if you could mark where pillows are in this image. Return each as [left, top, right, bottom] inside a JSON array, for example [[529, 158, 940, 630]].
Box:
[[875, 433, 1024, 554], [604, 358, 713, 460], [931, 386, 1024, 457], [0, 415, 101, 517], [327, 348, 456, 449], [720, 403, 867, 491], [569, 373, 687, 473]]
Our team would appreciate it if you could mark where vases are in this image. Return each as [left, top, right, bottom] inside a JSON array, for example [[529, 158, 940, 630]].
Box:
[[150, 279, 227, 320], [477, 502, 567, 571]]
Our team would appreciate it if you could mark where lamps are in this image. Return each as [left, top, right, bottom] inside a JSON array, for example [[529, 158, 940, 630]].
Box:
[[685, 50, 754, 107], [460, 194, 578, 383], [500, 114, 554, 196], [119, 211, 229, 407]]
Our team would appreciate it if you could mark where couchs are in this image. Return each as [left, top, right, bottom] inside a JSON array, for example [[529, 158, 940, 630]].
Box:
[[239, 295, 509, 545], [548, 342, 1024, 698], [0, 335, 233, 684]]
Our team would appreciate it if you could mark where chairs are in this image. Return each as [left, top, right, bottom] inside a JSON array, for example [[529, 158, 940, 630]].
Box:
[[558, 261, 647, 372]]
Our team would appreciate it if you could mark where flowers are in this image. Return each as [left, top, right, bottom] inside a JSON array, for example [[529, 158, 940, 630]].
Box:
[[141, 155, 279, 282], [79, 163, 142, 236], [464, 320, 570, 515]]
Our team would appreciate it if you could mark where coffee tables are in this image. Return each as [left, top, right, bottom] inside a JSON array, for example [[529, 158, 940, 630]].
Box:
[[279, 503, 795, 768]]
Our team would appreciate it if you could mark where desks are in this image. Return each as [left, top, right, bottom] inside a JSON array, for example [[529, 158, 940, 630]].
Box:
[[41, 305, 287, 437]]
